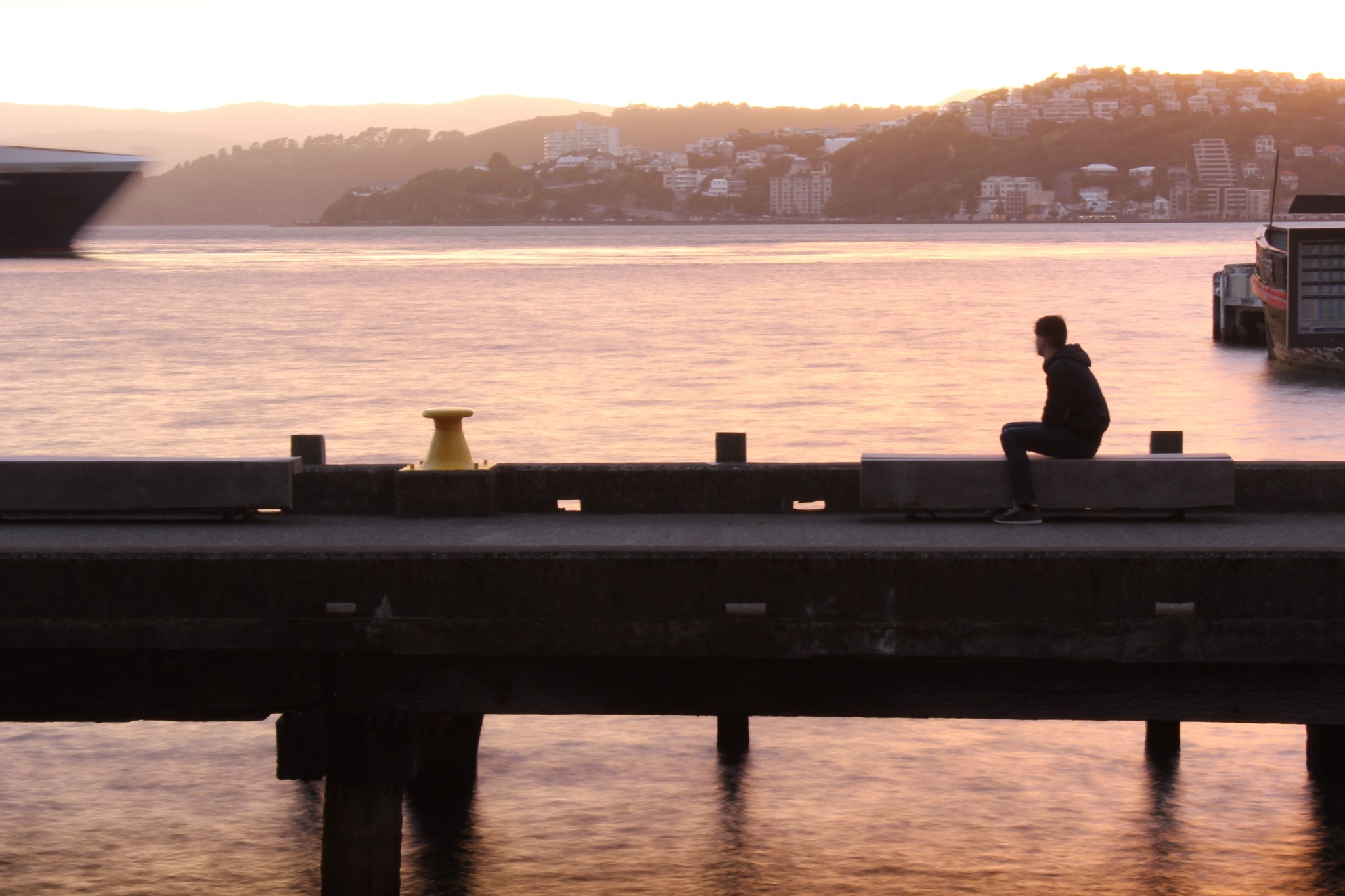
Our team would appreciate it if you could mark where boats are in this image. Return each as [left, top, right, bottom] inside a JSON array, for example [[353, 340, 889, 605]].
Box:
[[0, 146, 155, 260]]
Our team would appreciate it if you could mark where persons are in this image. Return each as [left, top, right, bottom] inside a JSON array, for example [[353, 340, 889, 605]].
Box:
[[991, 315, 1110, 525]]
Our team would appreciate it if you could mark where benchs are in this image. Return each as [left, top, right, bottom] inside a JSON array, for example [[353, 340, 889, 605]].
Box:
[[0, 456, 303, 511], [860, 453, 1233, 510]]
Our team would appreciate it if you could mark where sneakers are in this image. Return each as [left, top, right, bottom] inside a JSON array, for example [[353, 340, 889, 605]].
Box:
[[993, 504, 1042, 524]]
